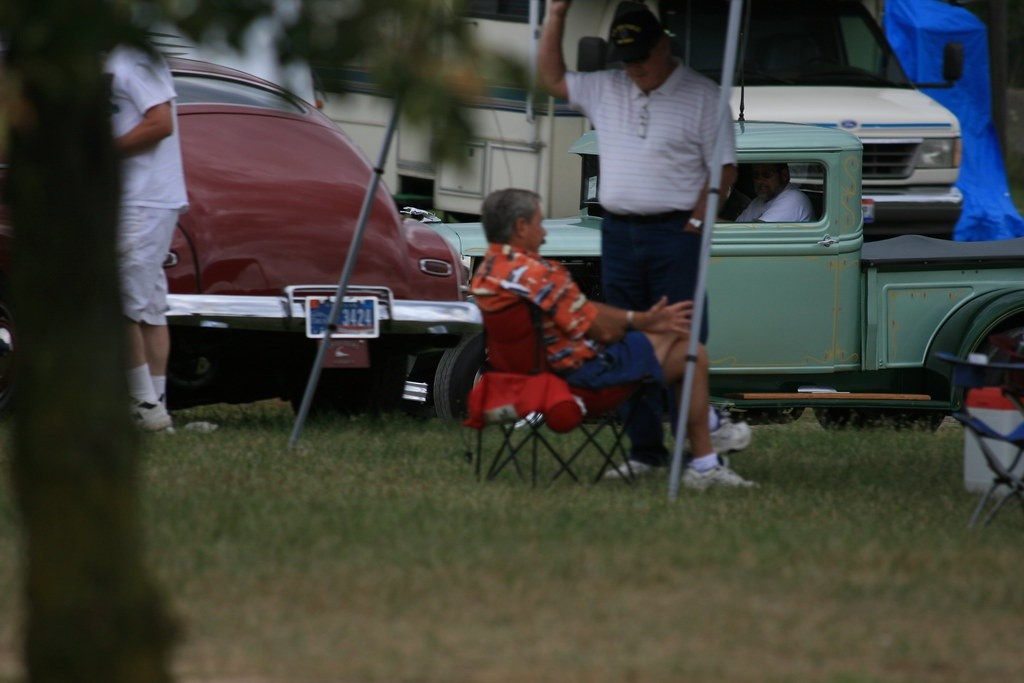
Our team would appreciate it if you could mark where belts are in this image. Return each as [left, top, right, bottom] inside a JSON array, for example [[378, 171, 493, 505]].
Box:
[[588, 204, 692, 224]]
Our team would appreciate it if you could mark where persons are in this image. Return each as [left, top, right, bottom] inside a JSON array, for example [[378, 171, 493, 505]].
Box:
[[536, 0, 736, 481], [105, 1, 190, 436], [735, 163, 813, 221], [468, 187, 760, 492]]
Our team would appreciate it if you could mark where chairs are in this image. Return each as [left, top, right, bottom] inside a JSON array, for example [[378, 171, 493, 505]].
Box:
[[468, 296, 637, 489], [933, 352, 1024, 530]]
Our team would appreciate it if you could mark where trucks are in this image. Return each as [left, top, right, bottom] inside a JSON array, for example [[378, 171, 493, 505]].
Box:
[[316, 0, 965, 253]]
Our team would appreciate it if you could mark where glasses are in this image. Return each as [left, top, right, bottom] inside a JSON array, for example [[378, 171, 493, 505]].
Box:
[[751, 169, 781, 181]]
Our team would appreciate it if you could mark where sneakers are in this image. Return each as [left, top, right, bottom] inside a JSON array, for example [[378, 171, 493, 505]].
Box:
[[681, 455, 760, 492], [701, 419, 751, 454], [128, 393, 173, 432], [605, 461, 666, 480]]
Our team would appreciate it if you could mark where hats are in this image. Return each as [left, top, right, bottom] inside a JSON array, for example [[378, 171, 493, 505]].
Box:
[[605, 9, 662, 62]]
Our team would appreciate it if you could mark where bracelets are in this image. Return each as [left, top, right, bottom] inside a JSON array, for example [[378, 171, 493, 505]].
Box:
[[689, 218, 704, 231], [626, 311, 634, 329]]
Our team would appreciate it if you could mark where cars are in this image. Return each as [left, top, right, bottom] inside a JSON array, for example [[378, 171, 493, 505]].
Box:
[[418, 127, 1024, 443], [161, 54, 484, 420]]
